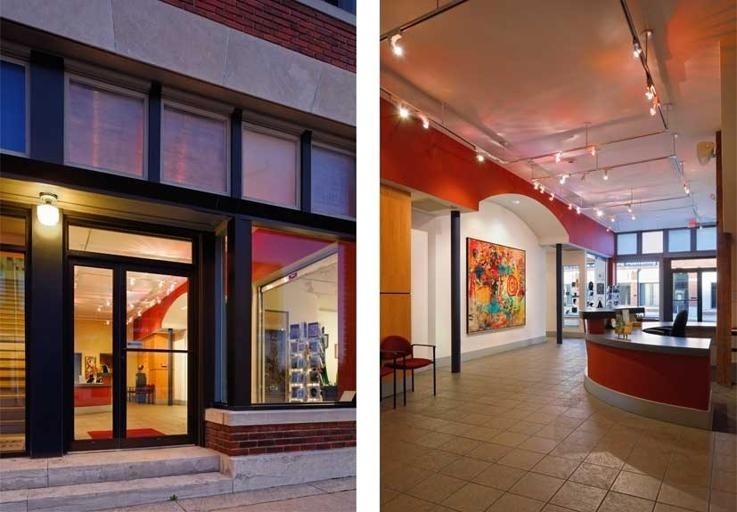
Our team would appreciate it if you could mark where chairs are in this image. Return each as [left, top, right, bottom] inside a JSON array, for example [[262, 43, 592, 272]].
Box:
[[664, 310, 691, 336], [379, 334, 441, 409]]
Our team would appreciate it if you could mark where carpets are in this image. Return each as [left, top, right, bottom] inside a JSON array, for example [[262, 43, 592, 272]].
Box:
[[88, 426, 164, 440]]
